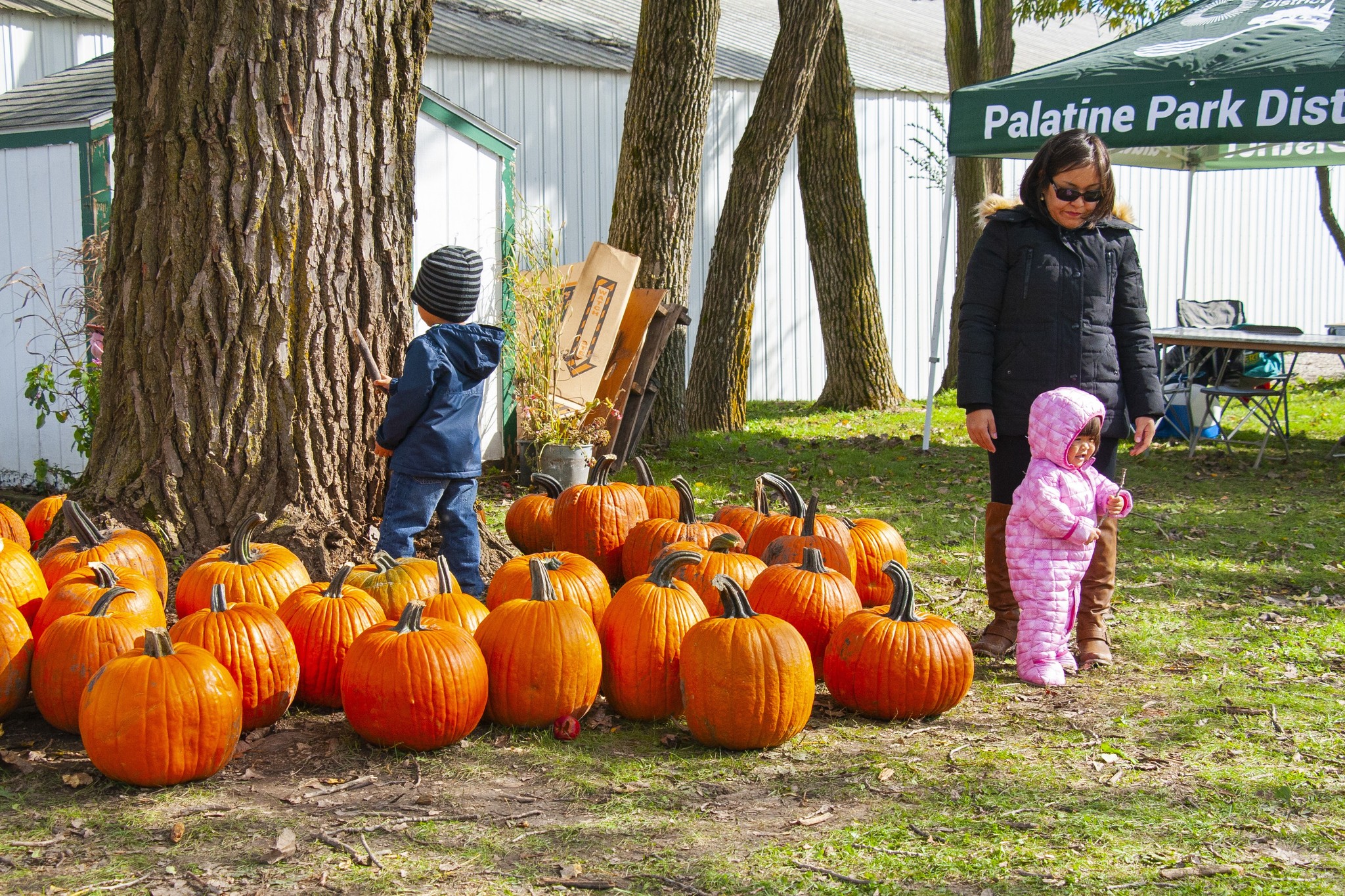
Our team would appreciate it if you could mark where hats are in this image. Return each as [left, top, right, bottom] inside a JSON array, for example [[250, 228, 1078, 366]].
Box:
[[411, 245, 483, 323]]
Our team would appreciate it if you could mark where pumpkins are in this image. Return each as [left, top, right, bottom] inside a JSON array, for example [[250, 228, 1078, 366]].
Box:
[[2, 453, 975, 788]]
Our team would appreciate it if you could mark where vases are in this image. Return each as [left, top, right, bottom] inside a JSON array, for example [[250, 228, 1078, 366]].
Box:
[[541, 442, 595, 489]]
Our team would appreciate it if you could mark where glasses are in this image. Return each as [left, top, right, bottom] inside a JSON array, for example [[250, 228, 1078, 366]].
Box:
[[1051, 178, 1106, 202]]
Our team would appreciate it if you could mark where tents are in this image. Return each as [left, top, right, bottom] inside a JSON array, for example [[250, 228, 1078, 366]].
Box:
[[922, 0, 1345, 452]]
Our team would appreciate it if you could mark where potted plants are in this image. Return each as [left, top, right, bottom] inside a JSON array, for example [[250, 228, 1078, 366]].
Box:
[[478, 183, 567, 486]]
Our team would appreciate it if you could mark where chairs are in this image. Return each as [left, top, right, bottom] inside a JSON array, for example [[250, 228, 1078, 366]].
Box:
[[1187, 324, 1306, 470], [1173, 294, 1292, 437]]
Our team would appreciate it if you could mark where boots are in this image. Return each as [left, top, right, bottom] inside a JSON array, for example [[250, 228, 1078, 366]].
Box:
[[1076, 514, 1118, 665], [972, 502, 1020, 659]]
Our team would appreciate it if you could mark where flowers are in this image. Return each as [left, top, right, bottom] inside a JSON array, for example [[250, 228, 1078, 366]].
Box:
[[522, 389, 624, 471]]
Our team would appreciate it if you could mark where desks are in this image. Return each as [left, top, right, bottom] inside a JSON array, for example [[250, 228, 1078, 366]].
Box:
[[1143, 325, 1345, 460]]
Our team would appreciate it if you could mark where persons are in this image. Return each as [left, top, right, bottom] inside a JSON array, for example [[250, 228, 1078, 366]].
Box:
[[1006, 386, 1133, 689], [956, 128, 1166, 669], [372, 245, 505, 601]]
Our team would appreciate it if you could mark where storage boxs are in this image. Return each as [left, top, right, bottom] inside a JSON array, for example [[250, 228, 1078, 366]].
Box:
[[1155, 382, 1222, 440]]
